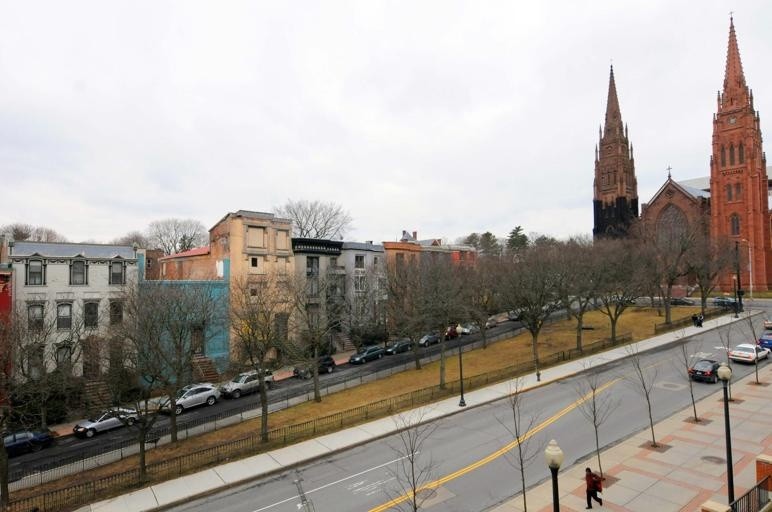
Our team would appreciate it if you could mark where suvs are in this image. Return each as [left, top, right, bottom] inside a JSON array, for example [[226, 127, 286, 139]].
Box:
[[221, 367, 276, 398], [293, 354, 335, 379], [712, 296, 735, 306]]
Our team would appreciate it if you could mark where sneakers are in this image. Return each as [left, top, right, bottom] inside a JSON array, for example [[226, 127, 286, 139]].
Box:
[[586, 498, 602, 509]]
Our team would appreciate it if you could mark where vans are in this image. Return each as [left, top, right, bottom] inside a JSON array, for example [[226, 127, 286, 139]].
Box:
[[670, 296, 696, 306]]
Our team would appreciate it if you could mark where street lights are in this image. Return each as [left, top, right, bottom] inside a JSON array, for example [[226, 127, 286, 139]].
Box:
[[742, 238, 753, 300], [716, 362, 737, 512], [456, 322, 467, 406], [542, 436, 566, 511], [733, 274, 739, 318]]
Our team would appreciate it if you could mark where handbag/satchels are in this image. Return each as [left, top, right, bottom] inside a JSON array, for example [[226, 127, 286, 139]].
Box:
[[596, 481, 602, 492]]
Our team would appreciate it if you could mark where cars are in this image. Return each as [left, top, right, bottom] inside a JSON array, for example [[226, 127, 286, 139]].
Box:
[[72, 405, 142, 437], [728, 314, 771, 365], [688, 357, 722, 384], [348, 313, 498, 365], [4, 426, 55, 455], [618, 296, 637, 305], [507, 297, 577, 321], [158, 382, 222, 415]]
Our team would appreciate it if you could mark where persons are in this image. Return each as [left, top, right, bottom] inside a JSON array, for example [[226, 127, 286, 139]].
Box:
[[585, 467, 602, 509]]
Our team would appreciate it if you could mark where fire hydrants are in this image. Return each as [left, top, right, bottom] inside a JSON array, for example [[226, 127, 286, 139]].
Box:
[[535, 370, 542, 381]]
